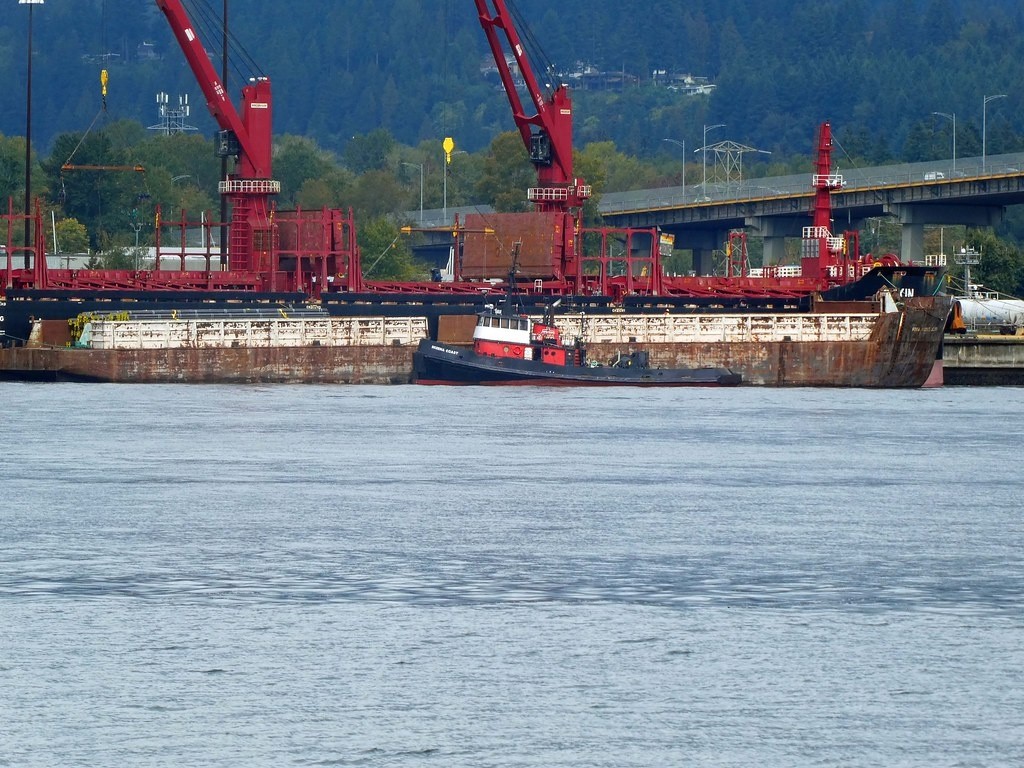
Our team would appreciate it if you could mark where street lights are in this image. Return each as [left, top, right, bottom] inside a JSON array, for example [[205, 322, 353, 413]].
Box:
[[703, 124, 727, 201], [982, 94, 1009, 174], [932, 112, 956, 173], [401, 162, 423, 227], [662, 137, 685, 203]]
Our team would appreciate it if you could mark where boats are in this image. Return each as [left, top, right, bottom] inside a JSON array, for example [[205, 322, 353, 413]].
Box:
[[410, 231, 965, 391]]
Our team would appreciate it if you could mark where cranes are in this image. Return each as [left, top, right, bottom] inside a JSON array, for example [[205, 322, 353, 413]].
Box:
[[153, 1, 284, 269], [472, 0, 591, 213]]
